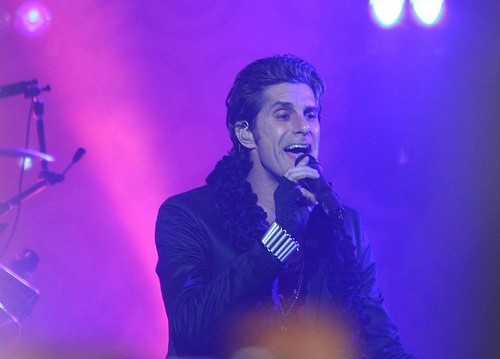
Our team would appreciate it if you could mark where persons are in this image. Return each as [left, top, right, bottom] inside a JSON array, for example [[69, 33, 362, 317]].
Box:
[[190, 299, 376, 359], [154, 51, 415, 359]]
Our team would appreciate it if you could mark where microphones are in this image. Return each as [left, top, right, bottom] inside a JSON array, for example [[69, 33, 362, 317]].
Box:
[[294, 152, 346, 231]]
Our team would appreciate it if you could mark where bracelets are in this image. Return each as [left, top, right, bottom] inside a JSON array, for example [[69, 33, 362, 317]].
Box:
[[260, 221, 301, 263]]
